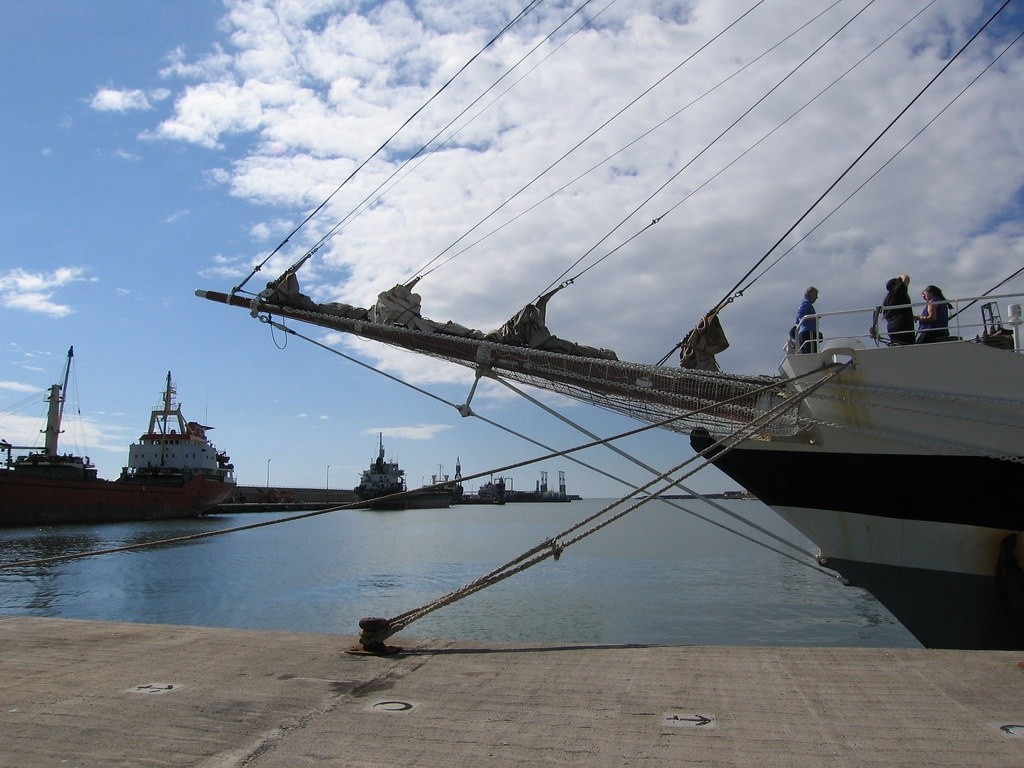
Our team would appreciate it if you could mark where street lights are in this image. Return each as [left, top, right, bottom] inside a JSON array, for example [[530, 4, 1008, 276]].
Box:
[[326, 465, 330, 490], [267, 458, 272, 487]]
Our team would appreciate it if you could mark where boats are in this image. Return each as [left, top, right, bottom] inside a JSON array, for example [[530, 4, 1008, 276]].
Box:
[[353, 430, 507, 511]]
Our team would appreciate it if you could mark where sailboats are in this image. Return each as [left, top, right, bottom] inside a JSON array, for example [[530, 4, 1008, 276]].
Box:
[[0, 341, 238, 523], [193, 1, 1024, 620]]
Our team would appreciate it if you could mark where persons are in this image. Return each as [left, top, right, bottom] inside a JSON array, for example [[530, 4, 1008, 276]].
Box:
[[796, 286, 819, 353], [883, 274, 954, 345]]
